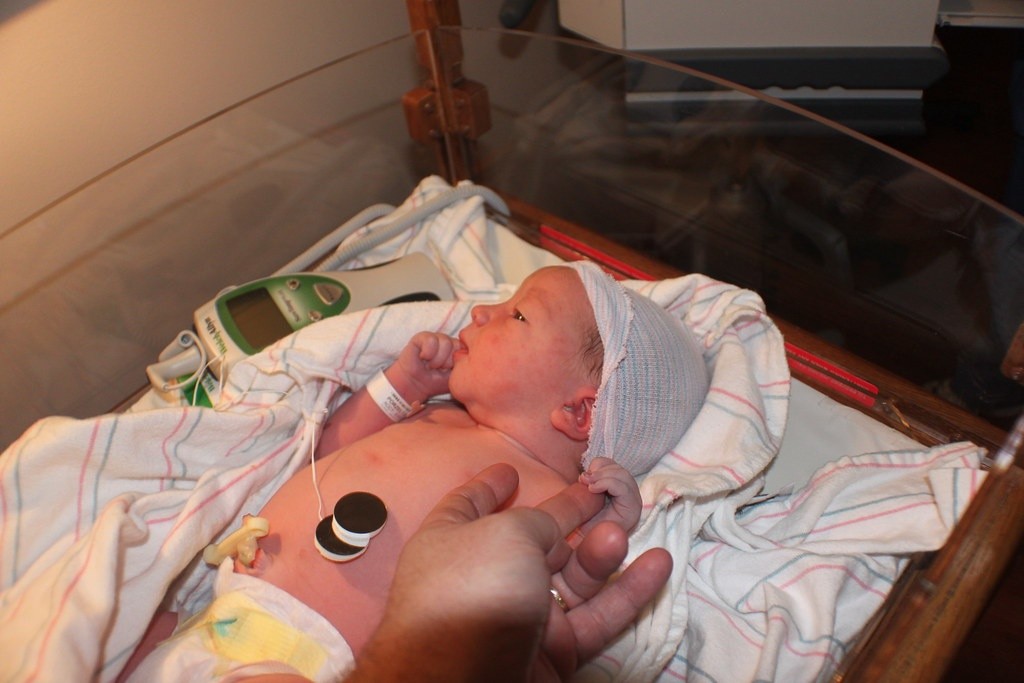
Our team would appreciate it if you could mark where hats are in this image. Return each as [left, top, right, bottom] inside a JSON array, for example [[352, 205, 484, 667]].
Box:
[[563, 223, 709, 476]]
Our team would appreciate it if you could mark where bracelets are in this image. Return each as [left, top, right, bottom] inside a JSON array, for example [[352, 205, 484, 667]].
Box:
[[360, 368, 411, 423]]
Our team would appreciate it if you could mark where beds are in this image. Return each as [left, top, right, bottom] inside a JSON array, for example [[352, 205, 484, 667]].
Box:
[[0, 0, 1023, 683]]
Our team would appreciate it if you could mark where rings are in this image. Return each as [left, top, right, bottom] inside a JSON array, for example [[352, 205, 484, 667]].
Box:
[[549, 585, 569, 613]]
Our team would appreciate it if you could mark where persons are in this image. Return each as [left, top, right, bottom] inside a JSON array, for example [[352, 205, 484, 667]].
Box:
[[114, 261, 708, 683]]
